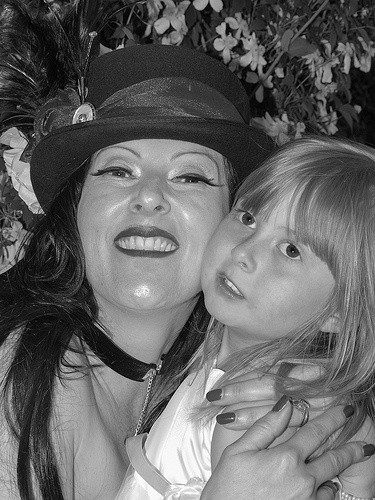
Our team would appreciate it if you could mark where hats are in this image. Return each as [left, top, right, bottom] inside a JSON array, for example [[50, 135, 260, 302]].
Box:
[[29, 44, 277, 208]]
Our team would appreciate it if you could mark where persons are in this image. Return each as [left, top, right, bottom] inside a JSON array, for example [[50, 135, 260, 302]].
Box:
[[0, 44, 375, 499], [114, 135, 375, 499]]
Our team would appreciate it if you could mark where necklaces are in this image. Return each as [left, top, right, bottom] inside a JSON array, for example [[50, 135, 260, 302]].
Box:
[[80, 311, 186, 439]]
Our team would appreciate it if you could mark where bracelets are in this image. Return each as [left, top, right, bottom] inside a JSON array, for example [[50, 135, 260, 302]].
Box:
[[337, 490, 368, 500]]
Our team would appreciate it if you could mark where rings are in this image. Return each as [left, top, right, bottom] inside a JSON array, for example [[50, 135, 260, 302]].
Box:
[[322, 480, 339, 496], [293, 397, 310, 427]]
